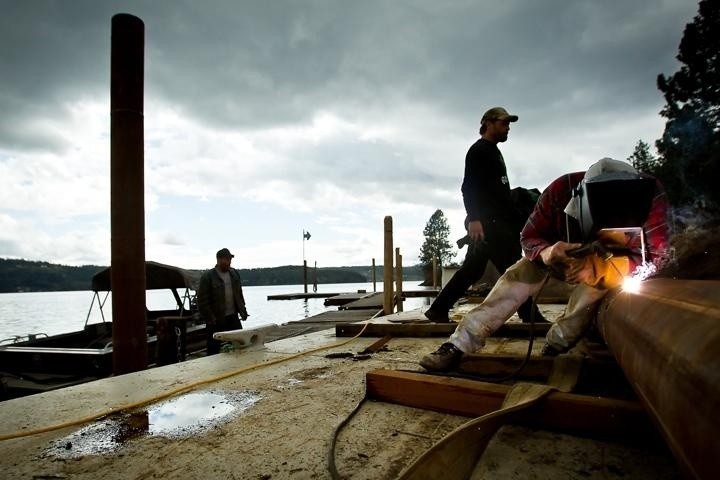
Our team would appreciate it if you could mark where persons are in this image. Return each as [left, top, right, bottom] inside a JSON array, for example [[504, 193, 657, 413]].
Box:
[[422, 103, 553, 326], [196, 246, 251, 357], [414, 156, 672, 376]]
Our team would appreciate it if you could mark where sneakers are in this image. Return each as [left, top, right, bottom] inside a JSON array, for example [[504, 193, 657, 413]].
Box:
[[425, 309, 449, 322], [419, 342, 462, 369]]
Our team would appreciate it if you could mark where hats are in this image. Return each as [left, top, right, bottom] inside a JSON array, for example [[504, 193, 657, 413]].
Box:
[[217, 248, 234, 258], [480, 108, 518, 124]]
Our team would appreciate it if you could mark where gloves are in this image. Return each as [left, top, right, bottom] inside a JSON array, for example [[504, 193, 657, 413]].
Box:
[[550, 257, 630, 289]]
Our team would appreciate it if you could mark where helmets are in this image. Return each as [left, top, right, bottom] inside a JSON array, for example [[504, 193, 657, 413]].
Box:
[[581, 159, 655, 232]]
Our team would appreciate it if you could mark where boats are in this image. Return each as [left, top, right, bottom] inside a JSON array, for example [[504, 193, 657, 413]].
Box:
[[1, 261, 208, 392]]
